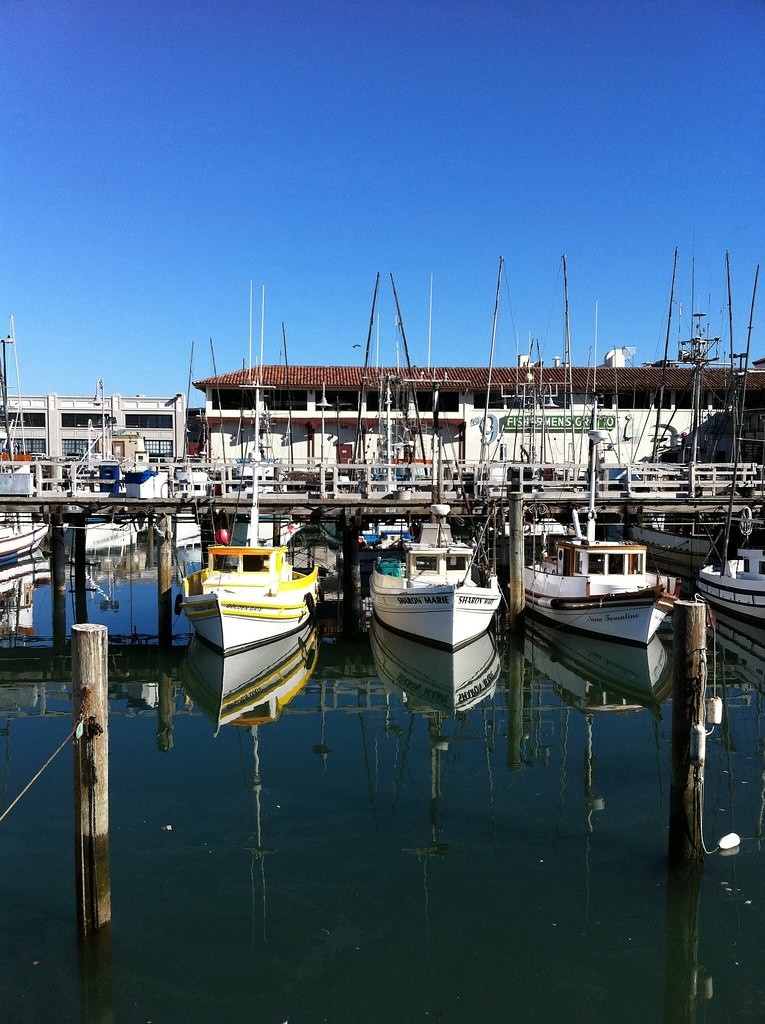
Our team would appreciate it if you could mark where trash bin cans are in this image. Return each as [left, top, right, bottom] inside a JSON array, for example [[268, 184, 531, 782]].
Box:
[[99, 461, 120, 493]]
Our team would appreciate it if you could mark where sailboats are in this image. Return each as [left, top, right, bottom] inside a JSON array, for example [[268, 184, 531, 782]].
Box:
[[0, 244, 764, 661]]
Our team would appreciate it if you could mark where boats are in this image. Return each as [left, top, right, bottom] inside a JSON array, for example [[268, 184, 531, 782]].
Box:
[[185, 621, 322, 738], [368, 613, 504, 719], [523, 616, 677, 716]]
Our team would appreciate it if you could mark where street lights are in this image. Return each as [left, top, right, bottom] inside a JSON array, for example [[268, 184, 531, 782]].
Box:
[[88, 377, 108, 460], [539, 383, 559, 491], [313, 381, 334, 497], [0, 336, 14, 411]]
